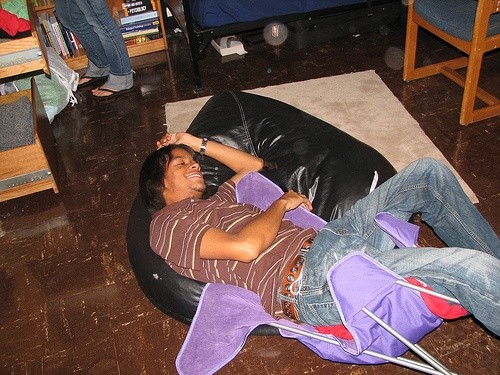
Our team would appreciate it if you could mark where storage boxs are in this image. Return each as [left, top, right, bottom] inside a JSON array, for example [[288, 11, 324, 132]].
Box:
[[0, 78, 60, 206], [0, 0, 50, 81]]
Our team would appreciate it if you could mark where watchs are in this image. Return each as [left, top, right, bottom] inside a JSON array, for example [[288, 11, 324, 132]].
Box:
[[200, 137, 209, 155]]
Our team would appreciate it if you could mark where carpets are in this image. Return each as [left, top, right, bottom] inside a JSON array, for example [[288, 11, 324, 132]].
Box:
[[164, 69, 480, 205]]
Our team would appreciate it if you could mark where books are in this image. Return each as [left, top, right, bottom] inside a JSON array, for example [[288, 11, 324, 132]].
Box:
[[109, 0, 163, 48], [32, 0, 83, 58]]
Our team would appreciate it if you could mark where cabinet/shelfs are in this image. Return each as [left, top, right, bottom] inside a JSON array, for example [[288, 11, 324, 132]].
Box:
[[28, 1, 169, 71]]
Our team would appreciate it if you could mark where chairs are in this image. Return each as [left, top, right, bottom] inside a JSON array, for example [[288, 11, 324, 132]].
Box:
[[403, 0, 499, 125]]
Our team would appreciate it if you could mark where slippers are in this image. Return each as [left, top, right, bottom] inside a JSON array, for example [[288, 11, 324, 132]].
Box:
[[90, 84, 135, 98], [77, 71, 109, 88]]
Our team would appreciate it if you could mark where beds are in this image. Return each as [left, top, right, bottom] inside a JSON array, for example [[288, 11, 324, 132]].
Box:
[[162, 0, 408, 90]]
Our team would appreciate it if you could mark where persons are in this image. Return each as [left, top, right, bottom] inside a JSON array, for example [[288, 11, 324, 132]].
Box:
[[53, 1, 136, 102], [138, 133, 500, 338]]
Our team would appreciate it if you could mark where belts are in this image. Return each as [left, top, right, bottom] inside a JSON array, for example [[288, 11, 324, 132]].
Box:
[[280, 237, 315, 320]]
